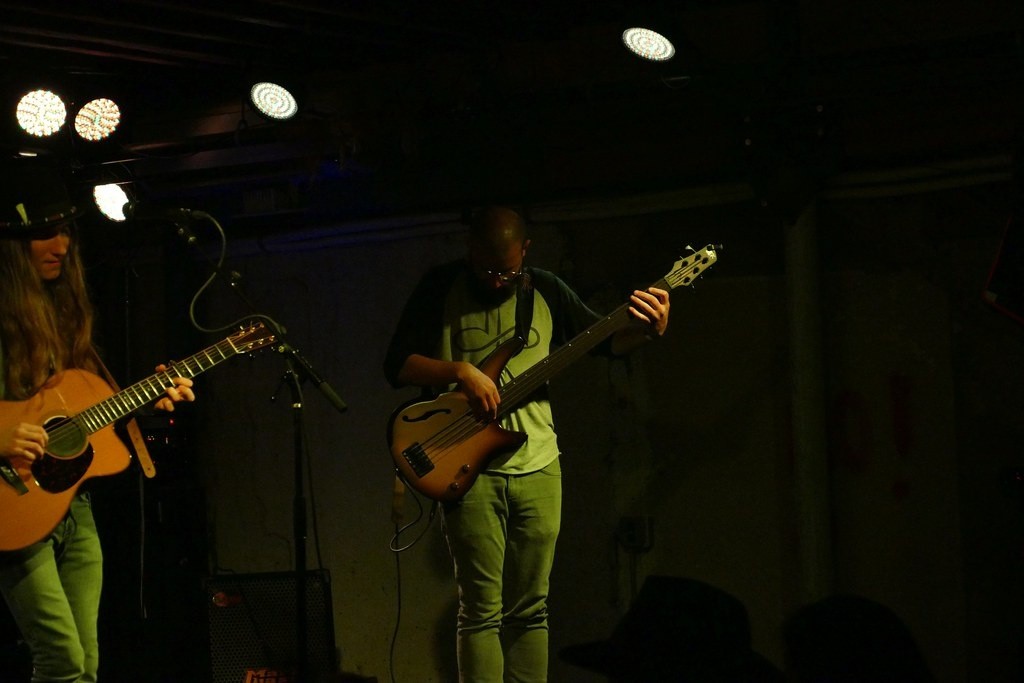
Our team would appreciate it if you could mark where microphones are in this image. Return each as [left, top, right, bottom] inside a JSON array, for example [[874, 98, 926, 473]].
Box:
[[123, 201, 207, 220]]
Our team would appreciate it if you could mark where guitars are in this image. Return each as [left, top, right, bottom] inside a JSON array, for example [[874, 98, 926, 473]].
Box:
[[386, 239, 721, 503], [1, 319, 278, 552]]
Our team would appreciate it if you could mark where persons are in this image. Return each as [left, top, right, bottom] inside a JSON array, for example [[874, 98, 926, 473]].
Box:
[[557, 575, 937, 683], [383, 207, 671, 683], [0, 183, 196, 683]]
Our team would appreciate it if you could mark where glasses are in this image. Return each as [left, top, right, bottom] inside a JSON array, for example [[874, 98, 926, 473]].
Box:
[[471, 250, 523, 281]]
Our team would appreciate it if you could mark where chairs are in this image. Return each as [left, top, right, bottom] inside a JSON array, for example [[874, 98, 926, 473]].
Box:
[[199, 569, 377, 683]]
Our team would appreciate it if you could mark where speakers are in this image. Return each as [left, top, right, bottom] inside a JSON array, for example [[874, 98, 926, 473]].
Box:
[[198, 568, 337, 683]]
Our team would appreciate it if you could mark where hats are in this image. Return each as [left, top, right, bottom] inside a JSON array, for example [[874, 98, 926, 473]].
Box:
[[557, 574, 790, 683], [0, 156, 88, 232]]
